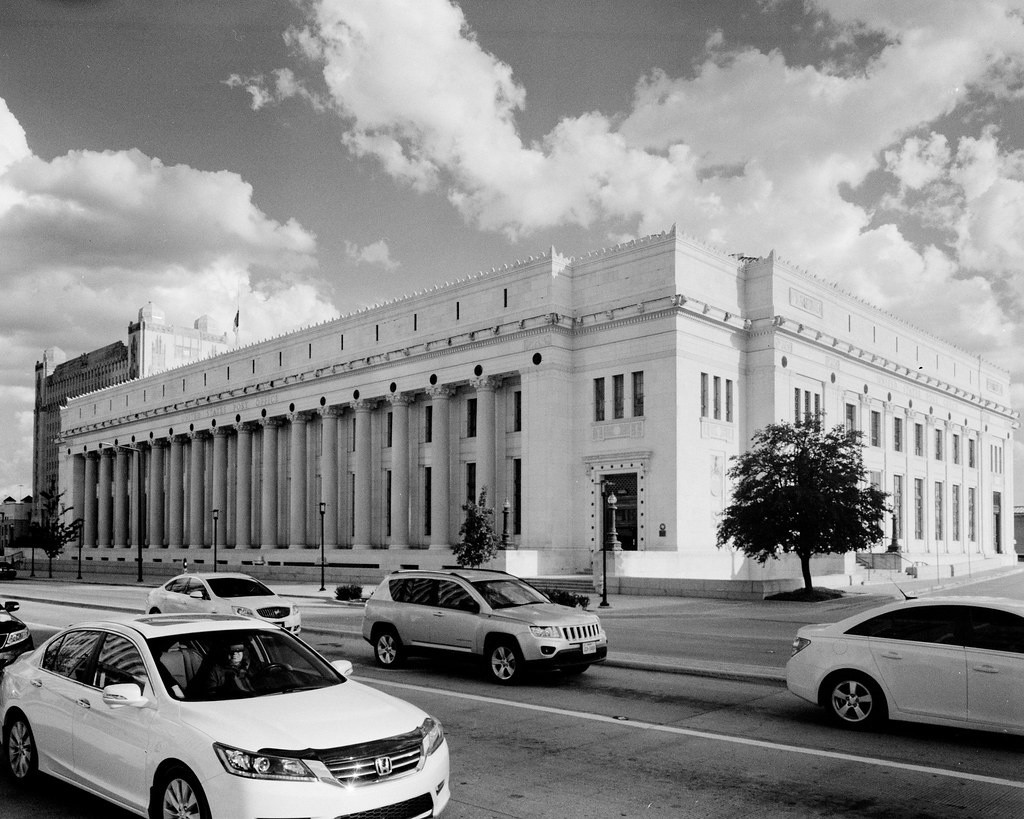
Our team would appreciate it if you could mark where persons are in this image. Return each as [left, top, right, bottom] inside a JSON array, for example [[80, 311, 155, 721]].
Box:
[[209, 642, 257, 695]]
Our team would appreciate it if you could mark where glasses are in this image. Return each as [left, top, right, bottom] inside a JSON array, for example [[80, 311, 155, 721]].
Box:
[[229, 644, 245, 653]]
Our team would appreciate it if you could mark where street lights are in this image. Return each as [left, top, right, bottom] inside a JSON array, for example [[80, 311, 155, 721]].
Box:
[[213, 509, 218, 573], [319, 502, 326, 592], [10, 523, 15, 547], [30, 521, 35, 577], [77, 518, 84, 579], [600, 479, 609, 606]]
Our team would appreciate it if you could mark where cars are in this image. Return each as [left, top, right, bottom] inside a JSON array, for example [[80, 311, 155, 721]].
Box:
[[151, 572, 302, 636], [786, 595, 1023, 738], [0, 611, 451, 818], [0, 600, 36, 676]]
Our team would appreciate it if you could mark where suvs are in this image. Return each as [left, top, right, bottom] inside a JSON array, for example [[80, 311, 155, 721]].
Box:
[[362, 568, 606, 687]]
